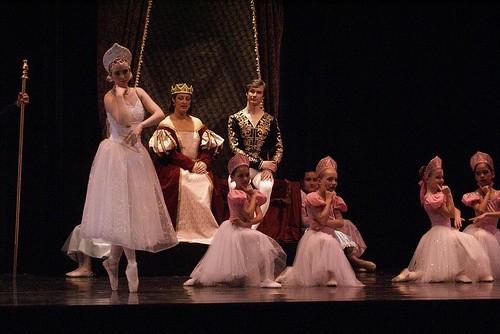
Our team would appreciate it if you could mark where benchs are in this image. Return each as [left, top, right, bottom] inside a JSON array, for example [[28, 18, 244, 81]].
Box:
[[215, 174, 302, 267]]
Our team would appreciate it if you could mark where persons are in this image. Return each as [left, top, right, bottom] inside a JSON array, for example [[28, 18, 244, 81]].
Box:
[[393, 167, 478, 283], [80, 58, 166, 293], [275, 167, 363, 287], [149, 83, 224, 246], [462, 161, 500, 281], [66, 223, 113, 278], [300, 170, 377, 273], [227, 79, 283, 231], [183, 162, 283, 289]]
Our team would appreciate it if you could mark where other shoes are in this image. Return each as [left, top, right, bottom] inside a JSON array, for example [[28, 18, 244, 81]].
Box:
[[391, 269, 411, 282], [103, 257, 118, 291], [456, 274, 472, 283], [125, 261, 140, 292], [66, 267, 93, 277], [327, 278, 338, 286], [183, 279, 195, 286], [259, 278, 282, 288], [479, 276, 494, 282]]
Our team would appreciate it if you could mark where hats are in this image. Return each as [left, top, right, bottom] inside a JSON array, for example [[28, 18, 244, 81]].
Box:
[[228, 153, 250, 175], [422, 156, 442, 181], [469, 151, 494, 173], [103, 43, 132, 72], [316, 156, 337, 178]]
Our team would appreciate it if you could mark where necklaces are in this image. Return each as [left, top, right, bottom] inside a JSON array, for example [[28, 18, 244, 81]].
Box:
[[113, 86, 131, 95]]
